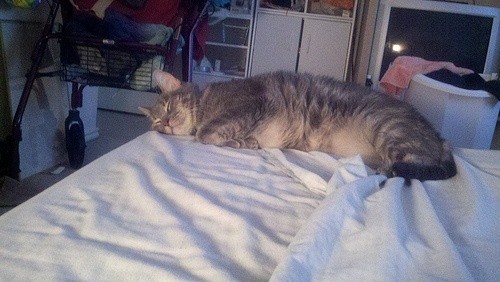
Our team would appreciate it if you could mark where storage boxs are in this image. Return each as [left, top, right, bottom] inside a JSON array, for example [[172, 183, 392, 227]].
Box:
[[405, 74, 500, 149]]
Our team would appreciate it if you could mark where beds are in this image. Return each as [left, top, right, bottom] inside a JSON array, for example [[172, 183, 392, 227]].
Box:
[[0, 132, 500, 282]]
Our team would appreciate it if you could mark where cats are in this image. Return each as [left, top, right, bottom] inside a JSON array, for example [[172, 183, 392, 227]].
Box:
[[137, 68, 458, 181]]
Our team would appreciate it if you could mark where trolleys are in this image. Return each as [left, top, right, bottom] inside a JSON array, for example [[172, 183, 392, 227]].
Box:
[[0, 1, 216, 182]]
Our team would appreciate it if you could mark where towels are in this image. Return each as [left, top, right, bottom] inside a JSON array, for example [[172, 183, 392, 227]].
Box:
[[267, 153, 496, 282], [379, 56, 473, 94]]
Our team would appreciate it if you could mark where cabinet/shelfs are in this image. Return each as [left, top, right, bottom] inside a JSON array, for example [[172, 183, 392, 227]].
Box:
[[192, 0, 358, 84]]
